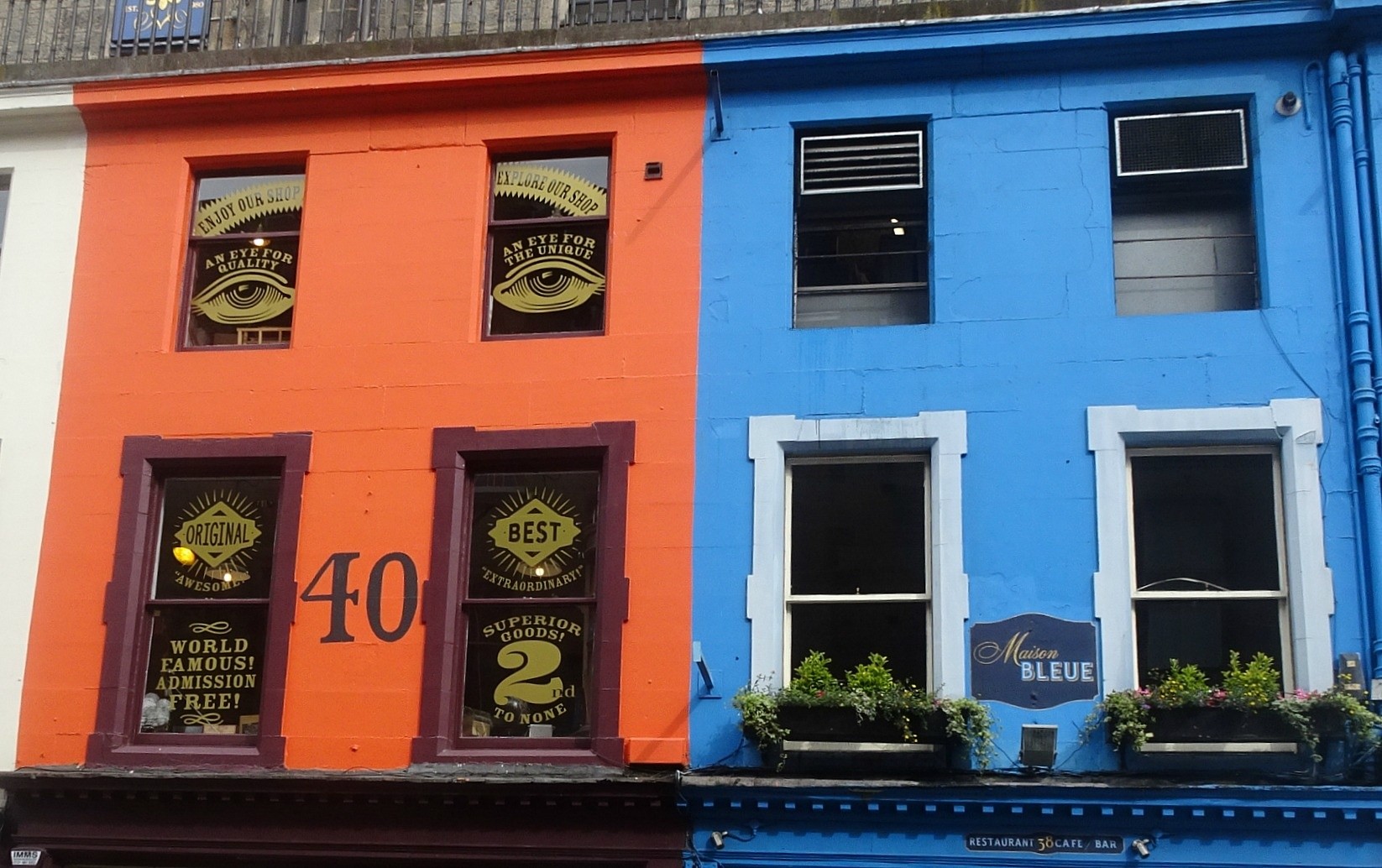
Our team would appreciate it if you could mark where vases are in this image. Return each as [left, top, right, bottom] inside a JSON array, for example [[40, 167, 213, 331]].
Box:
[[1138, 706, 1285, 741], [777, 705, 970, 745]]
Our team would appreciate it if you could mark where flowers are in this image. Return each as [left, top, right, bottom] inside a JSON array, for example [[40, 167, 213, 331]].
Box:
[[731, 648, 998, 774], [1079, 649, 1382, 749]]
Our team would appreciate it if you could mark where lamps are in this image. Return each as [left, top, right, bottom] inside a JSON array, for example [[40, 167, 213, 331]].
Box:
[[205, 561, 251, 582], [1132, 839, 1150, 859], [878, 191, 912, 236], [517, 552, 562, 577], [711, 831, 728, 849], [1018, 723, 1058, 773], [248, 217, 272, 247]]
